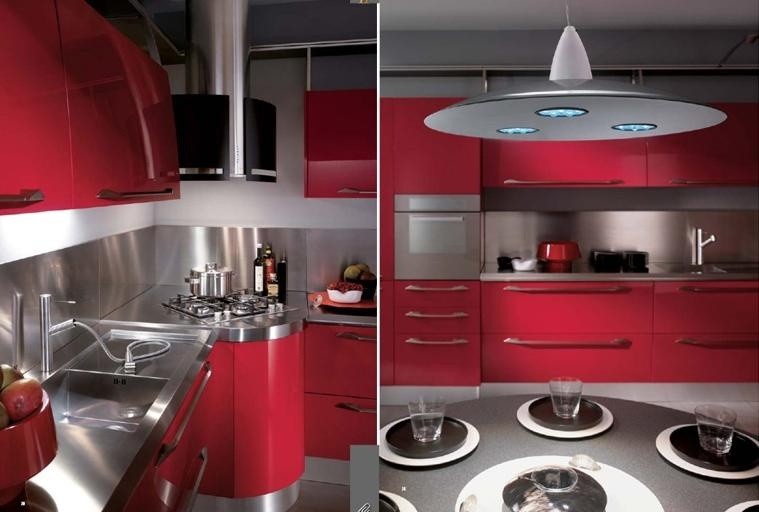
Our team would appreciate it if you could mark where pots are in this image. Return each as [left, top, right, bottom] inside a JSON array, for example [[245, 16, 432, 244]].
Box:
[[185, 262, 237, 297]]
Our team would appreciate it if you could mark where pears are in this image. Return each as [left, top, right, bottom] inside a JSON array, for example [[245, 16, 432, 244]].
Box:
[[343, 264, 360, 280], [356, 263, 369, 273]]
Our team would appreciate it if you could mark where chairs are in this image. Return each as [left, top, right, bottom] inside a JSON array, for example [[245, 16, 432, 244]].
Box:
[[375, 394, 759, 512]]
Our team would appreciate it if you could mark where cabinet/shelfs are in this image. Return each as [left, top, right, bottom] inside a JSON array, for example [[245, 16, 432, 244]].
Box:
[[654, 272, 759, 403], [395, 98, 480, 194], [481, 272, 654, 401], [648, 103, 759, 188], [305, 317, 383, 486], [481, 134, 648, 187], [307, 90, 378, 199], [0, 1, 180, 213], [394, 281, 483, 403], [125, 340, 215, 510]]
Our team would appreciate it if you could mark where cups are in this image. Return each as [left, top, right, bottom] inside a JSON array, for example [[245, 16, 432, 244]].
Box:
[[694, 403, 737, 455], [407, 396, 445, 442], [549, 376, 583, 419]]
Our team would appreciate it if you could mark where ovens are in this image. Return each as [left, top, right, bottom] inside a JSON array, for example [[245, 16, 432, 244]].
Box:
[[393, 194, 485, 280]]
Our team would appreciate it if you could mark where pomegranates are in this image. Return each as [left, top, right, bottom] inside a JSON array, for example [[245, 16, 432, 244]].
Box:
[[0, 363, 24, 393], [0, 403, 8, 430], [0, 365, 5, 393], [0, 378, 44, 421]]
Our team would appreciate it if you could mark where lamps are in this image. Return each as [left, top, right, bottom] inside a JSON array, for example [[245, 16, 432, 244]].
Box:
[[422, 1, 728, 140]]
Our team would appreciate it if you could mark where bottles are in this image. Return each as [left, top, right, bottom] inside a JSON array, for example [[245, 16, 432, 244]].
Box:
[[267, 273, 279, 303], [277, 248, 290, 305], [253, 244, 265, 296], [263, 242, 275, 296]]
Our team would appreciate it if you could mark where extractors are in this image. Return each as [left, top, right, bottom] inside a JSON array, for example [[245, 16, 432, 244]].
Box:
[[171, 3, 277, 183]]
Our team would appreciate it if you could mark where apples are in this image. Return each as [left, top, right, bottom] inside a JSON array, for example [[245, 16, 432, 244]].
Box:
[[360, 272, 376, 281]]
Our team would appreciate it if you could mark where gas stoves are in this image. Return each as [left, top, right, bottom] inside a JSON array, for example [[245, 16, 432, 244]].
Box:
[[162, 290, 299, 325]]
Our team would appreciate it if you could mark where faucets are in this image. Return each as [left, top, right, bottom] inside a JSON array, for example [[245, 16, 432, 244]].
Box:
[[39, 291, 77, 372], [0, 292, 24, 369], [689, 226, 714, 268]]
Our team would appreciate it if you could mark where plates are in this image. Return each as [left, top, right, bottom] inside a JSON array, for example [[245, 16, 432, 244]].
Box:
[[385, 415, 467, 459], [379, 489, 418, 511], [669, 424, 759, 472], [307, 290, 374, 309], [529, 395, 603, 431], [741, 505, 758, 512], [501, 467, 607, 512], [655, 421, 759, 480], [379, 493, 400, 512], [379, 413, 480, 468], [723, 500, 759, 512], [516, 396, 614, 439]]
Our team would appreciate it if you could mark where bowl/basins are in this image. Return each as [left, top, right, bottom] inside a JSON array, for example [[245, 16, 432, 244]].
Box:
[[513, 259, 538, 271], [455, 456, 665, 512], [327, 289, 363, 304], [497, 256, 521, 269]]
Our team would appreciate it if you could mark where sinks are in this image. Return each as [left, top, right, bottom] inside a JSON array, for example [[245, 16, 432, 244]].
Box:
[[39, 363, 170, 438], [715, 261, 759, 274]]
